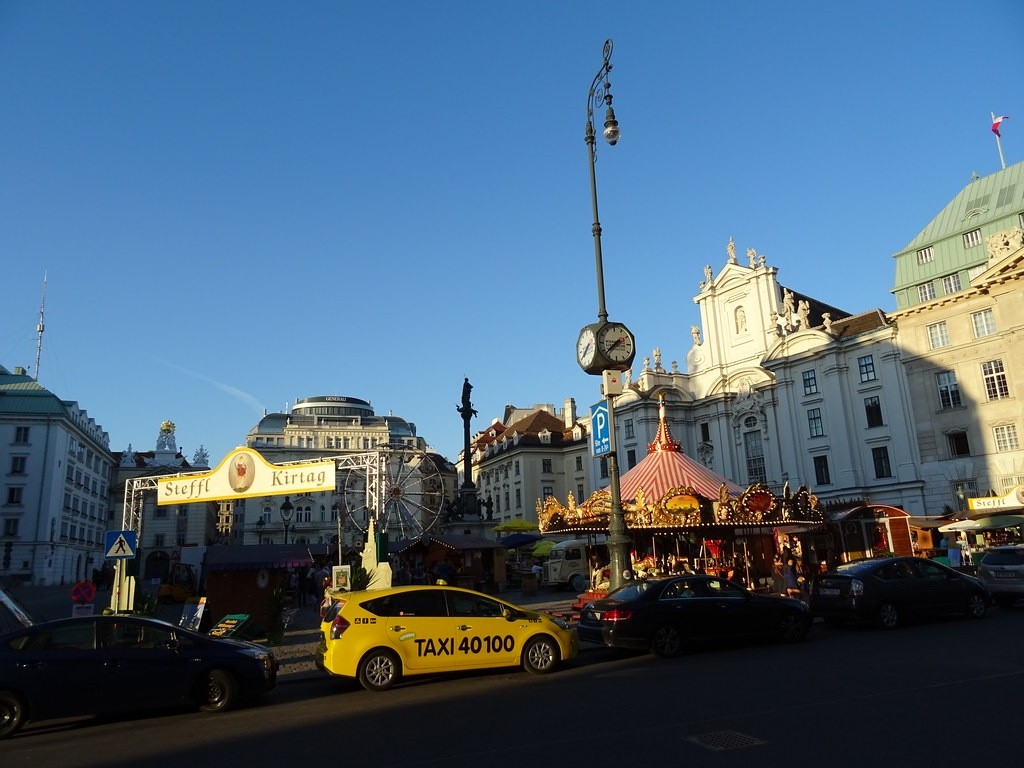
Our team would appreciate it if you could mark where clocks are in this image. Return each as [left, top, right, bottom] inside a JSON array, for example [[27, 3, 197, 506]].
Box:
[[577, 322, 635, 370]]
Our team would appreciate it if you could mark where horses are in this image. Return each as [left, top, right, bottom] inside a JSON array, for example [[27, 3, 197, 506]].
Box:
[[630, 551, 660, 579]]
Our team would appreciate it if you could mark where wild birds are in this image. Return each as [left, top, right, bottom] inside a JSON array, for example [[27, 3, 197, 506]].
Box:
[[971, 170, 979, 181]]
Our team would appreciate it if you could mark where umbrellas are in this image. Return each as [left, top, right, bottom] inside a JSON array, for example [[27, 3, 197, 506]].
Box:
[[507, 549, 515, 553], [499, 533, 546, 562], [937, 515, 1024, 532], [525, 532, 546, 539], [531, 540, 557, 557], [490, 518, 539, 531]]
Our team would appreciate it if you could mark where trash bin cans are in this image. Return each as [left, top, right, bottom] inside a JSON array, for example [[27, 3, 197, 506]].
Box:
[[521, 574, 535, 598]]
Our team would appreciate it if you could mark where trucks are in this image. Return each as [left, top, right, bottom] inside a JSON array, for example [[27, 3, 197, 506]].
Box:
[[549, 539, 590, 591]]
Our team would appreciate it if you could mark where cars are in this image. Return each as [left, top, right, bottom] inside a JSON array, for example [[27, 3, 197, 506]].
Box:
[[576, 572, 815, 657], [809, 555, 994, 630], [315, 579, 580, 691], [0, 614, 280, 740]]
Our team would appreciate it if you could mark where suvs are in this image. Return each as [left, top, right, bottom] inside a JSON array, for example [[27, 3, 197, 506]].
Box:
[[976, 543, 1024, 609]]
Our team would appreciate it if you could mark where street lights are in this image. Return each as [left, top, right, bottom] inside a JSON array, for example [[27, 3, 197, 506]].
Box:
[[279, 496, 295, 543], [585, 38, 637, 596], [256, 515, 266, 544], [289, 524, 296, 544]]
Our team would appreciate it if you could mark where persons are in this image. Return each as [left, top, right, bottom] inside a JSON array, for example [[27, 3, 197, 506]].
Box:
[[483, 495, 493, 520], [444, 498, 459, 522], [731, 552, 746, 584], [509, 554, 515, 562], [679, 586, 695, 598], [939, 535, 949, 557], [780, 559, 798, 592], [531, 556, 547, 586], [826, 546, 834, 569], [590, 561, 610, 590], [291, 560, 335, 612], [667, 552, 676, 572], [397, 557, 449, 586], [955, 536, 973, 566]]
